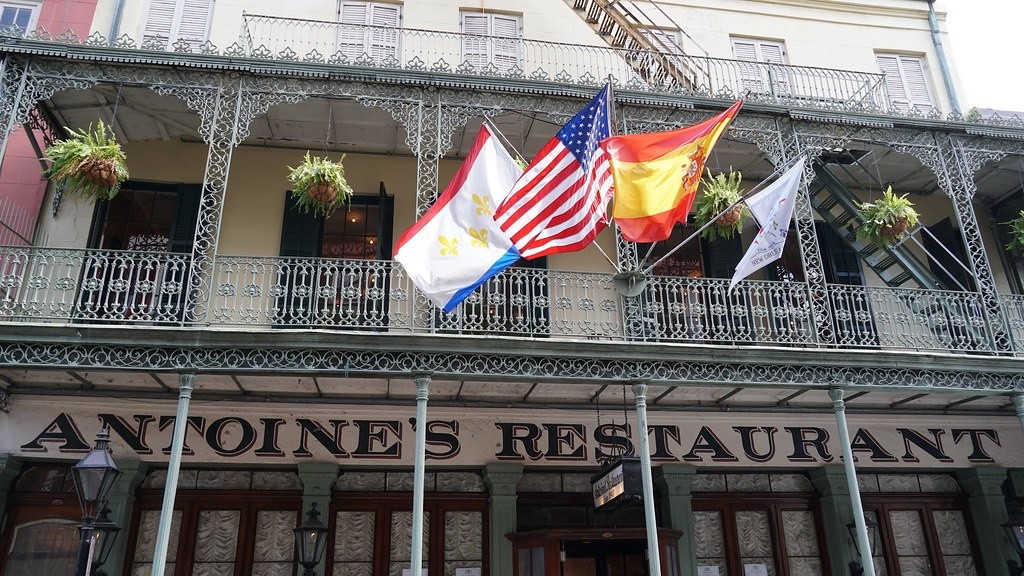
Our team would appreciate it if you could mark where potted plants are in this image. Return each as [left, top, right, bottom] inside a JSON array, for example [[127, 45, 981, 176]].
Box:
[[39, 117, 130, 204], [841, 185, 922, 251], [692, 165, 752, 243], [286, 150, 354, 221], [997, 210, 1024, 250]]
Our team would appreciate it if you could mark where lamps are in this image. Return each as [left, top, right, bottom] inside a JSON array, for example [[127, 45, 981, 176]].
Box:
[[61, 499, 123, 576], [291, 502, 329, 576], [1000, 496, 1024, 576], [846, 516, 880, 576]]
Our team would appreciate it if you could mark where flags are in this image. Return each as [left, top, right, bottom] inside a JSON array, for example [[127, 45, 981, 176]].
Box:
[[393, 124, 524, 314], [600, 100, 743, 242], [727, 152, 807, 296], [493, 83, 614, 261]]
